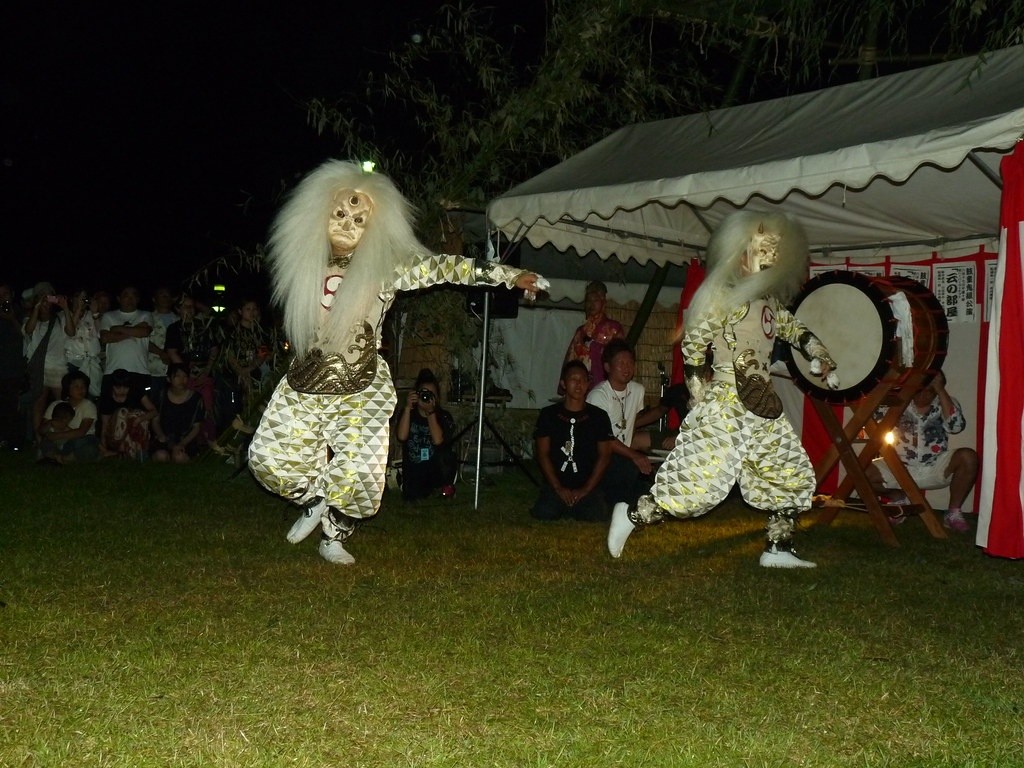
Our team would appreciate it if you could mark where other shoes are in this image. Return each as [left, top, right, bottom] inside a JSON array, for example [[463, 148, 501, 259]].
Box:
[[758, 550, 819, 571], [285, 497, 327, 546], [606, 501, 638, 559], [318, 537, 355, 567], [442, 484, 456, 498]]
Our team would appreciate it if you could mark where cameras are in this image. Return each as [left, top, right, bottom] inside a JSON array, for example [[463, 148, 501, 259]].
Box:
[[415, 388, 434, 401], [47, 296, 58, 303], [83, 296, 92, 305]]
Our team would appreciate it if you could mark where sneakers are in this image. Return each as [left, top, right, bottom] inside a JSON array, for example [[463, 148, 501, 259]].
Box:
[[944, 503, 969, 534], [880, 494, 906, 527]]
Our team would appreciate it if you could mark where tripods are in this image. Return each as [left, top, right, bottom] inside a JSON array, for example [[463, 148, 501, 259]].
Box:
[[451, 319, 535, 482]]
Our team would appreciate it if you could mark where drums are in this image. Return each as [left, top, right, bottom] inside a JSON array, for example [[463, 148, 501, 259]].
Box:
[[785, 271, 953, 406]]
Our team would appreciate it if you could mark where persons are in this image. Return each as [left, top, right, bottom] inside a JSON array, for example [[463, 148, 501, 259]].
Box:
[[395, 369, 457, 502], [247, 161, 540, 564], [529, 282, 714, 523], [864, 370, 979, 535], [609, 210, 838, 570], [0, 281, 277, 464]]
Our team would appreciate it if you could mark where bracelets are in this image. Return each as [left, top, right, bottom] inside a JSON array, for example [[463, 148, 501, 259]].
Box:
[[426, 411, 434, 416]]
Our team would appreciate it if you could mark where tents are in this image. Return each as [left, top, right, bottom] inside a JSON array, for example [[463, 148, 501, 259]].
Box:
[[474, 45, 1024, 511]]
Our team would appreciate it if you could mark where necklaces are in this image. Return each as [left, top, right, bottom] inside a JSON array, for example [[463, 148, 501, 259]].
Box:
[[612, 387, 626, 426]]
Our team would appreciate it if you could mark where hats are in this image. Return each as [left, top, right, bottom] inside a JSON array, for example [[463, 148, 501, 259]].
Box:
[[32, 282, 56, 304]]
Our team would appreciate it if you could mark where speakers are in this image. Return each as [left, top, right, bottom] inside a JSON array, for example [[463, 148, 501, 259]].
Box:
[[465, 241, 521, 319]]
